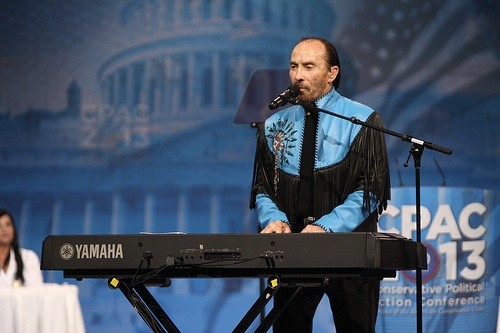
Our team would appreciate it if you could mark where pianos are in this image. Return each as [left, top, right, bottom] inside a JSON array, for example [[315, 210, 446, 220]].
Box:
[[38, 231, 428, 273]]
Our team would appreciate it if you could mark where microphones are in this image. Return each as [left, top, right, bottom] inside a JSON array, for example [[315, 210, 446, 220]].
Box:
[[269, 85, 300, 110]]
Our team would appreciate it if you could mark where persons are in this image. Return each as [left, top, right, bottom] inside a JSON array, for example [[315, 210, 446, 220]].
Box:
[[0, 208, 44, 283], [250, 38, 392, 333]]
